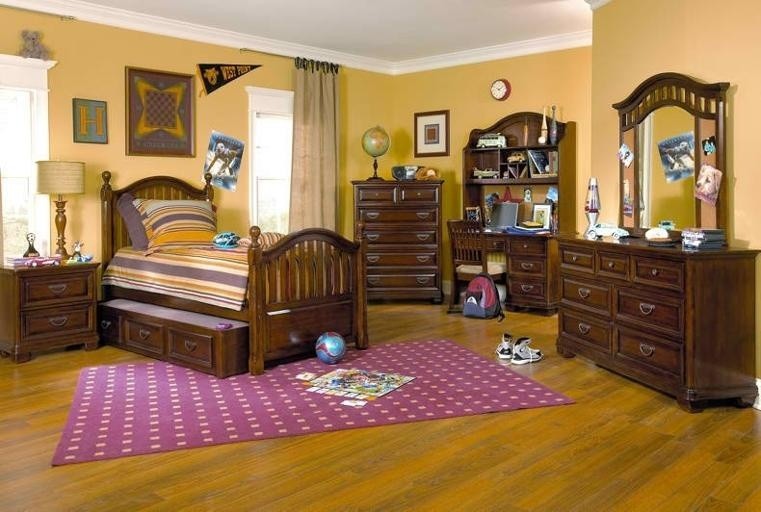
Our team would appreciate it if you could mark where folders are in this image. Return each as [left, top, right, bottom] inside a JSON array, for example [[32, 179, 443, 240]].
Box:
[[506, 223, 537, 236]]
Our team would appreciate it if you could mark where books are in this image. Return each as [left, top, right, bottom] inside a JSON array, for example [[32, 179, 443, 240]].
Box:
[[680, 227, 728, 252], [528, 148, 547, 173]]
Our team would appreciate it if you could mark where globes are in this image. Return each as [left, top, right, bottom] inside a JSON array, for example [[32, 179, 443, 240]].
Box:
[[361, 125, 391, 182]]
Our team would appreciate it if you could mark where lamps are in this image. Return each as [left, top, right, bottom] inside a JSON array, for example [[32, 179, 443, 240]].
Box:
[[584, 178, 600, 237], [36, 161, 86, 259]]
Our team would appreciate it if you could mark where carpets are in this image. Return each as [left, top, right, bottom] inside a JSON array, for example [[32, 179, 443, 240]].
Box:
[[50, 336, 579, 468]]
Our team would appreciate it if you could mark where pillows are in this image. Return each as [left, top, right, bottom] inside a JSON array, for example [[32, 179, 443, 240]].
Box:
[[116, 192, 148, 251], [134, 198, 221, 256], [236, 232, 289, 250]]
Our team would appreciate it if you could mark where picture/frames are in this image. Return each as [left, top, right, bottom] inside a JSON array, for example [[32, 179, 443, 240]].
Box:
[[413, 109, 450, 157], [611, 72, 731, 239], [124, 65, 196, 158], [73, 98, 108, 144]]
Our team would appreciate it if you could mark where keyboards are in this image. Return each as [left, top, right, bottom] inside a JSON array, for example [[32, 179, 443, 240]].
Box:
[[476, 229, 492, 233]]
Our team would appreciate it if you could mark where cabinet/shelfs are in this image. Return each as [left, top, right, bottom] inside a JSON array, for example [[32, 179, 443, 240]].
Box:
[[552, 234, 757, 413], [125, 318, 164, 353], [456, 106, 577, 315], [94, 313, 122, 343], [168, 328, 214, 368], [349, 177, 443, 306]]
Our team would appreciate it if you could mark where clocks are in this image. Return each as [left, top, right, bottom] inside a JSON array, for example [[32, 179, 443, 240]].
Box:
[[490, 79, 511, 100]]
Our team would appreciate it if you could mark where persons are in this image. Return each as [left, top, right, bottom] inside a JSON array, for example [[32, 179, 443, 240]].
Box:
[[206, 141, 237, 178], [702, 136, 717, 153]]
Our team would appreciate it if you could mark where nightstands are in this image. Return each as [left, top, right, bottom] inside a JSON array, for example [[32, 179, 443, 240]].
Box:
[[1, 260, 100, 363]]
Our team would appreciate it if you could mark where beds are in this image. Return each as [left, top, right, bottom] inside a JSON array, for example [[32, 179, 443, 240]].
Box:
[[101, 170, 369, 380]]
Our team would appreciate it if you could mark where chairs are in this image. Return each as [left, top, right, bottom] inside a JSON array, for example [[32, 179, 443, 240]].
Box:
[[446, 220, 507, 314]]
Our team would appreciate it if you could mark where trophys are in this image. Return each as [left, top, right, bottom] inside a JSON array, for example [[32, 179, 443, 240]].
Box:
[[22, 233, 41, 257]]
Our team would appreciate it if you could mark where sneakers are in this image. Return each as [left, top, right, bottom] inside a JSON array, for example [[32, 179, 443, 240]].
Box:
[[510, 337, 544, 365], [495, 332, 514, 360]]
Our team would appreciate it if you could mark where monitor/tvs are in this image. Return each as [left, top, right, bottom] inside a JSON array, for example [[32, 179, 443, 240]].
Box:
[[489, 202, 518, 228]]
